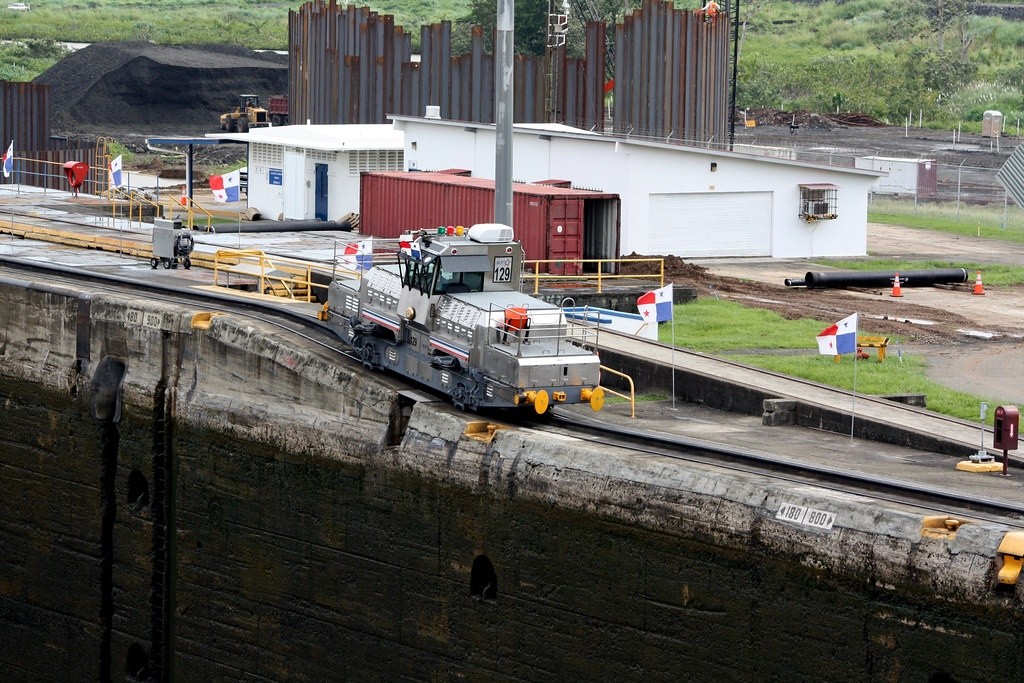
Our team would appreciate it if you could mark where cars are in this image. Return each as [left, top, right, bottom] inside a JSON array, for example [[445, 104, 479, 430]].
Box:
[[240, 167, 248, 195]]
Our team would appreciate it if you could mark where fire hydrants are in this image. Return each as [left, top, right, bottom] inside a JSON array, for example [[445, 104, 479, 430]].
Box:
[[855, 348, 870, 359]]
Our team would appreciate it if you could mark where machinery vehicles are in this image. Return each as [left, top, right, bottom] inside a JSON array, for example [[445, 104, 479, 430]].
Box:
[[268, 93, 290, 127], [216, 93, 272, 133]]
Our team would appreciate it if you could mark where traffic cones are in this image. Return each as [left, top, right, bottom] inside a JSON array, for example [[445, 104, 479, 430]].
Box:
[[971, 271, 986, 295], [889, 272, 904, 297]]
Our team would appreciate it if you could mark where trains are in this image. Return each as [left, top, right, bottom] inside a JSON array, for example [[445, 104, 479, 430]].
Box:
[[317, 221, 606, 419]]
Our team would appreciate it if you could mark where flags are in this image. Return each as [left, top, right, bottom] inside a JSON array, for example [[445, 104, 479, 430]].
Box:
[[401, 241, 420, 257], [637, 282, 672, 323], [108, 154, 122, 188], [344, 237, 371, 270], [815, 312, 856, 354], [3, 143, 12, 178], [209, 170, 240, 202]]
